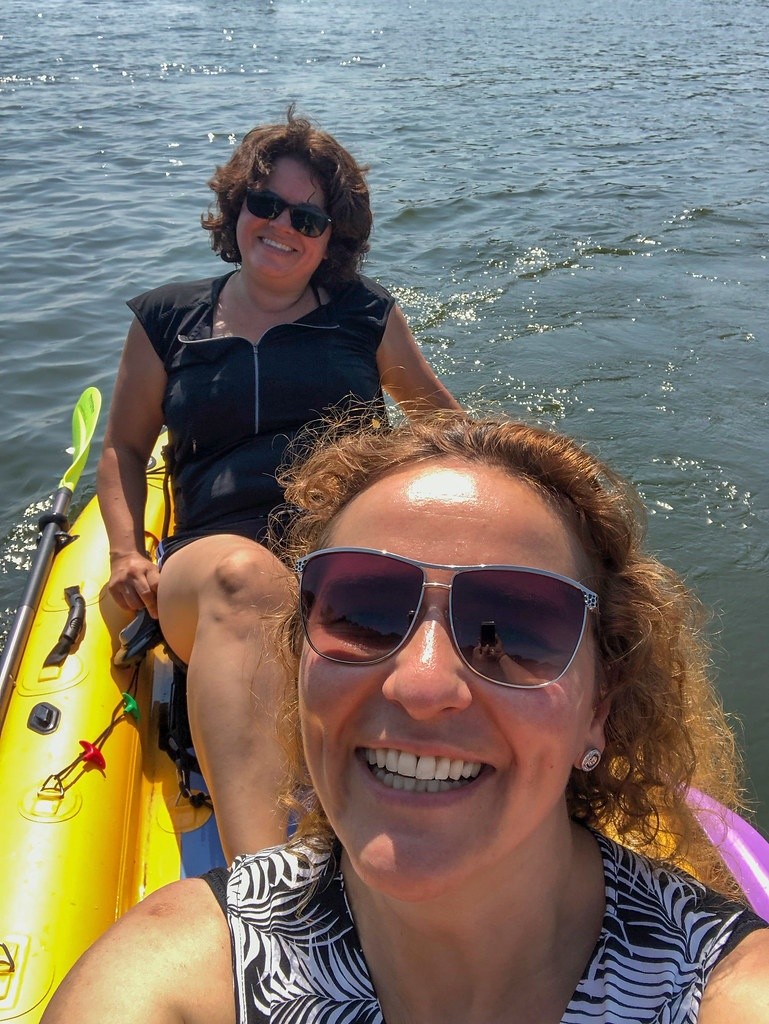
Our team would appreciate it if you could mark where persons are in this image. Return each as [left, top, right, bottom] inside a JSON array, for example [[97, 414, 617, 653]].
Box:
[[100, 103, 462, 868], [42, 402, 769, 1024]]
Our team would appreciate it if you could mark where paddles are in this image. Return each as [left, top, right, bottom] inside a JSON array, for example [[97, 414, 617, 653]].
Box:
[[656, 767, 769, 926], [0, 386, 102, 740]]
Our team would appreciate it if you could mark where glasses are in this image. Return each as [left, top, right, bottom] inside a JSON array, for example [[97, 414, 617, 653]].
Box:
[[245, 186, 333, 237], [295, 546, 601, 689]]
